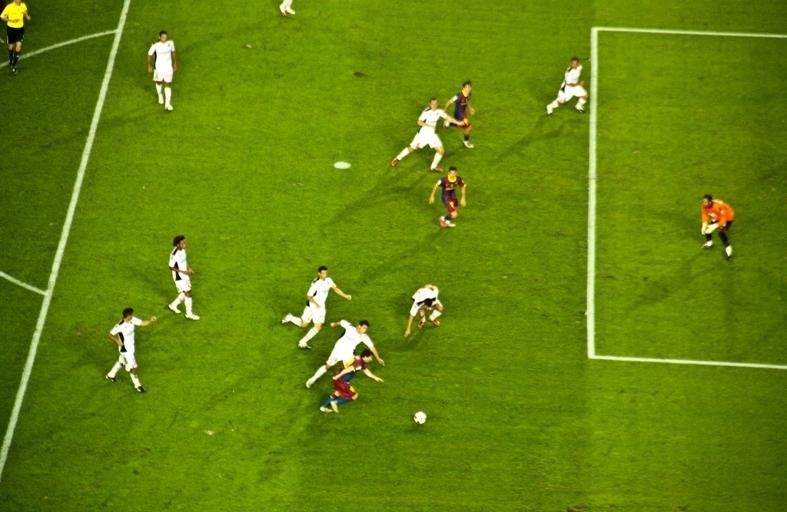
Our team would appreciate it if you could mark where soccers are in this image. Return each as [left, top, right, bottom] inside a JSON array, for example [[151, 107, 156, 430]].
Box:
[[414, 411, 427, 425]]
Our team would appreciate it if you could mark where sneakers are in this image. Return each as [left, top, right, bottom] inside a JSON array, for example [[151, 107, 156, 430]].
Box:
[[134, 385, 145, 392], [106, 375, 117, 383]]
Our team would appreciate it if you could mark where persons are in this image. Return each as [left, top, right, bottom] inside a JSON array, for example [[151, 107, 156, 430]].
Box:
[[279, 0, 296, 16], [701, 195, 735, 261], [147, 31, 177, 111], [320, 349, 384, 414], [443, 81, 475, 149], [391, 98, 465, 173], [305, 318, 385, 388], [282, 265, 352, 350], [428, 167, 467, 228], [404, 284, 443, 339], [168, 235, 201, 321], [105, 308, 157, 393], [0, 0, 33, 75], [546, 57, 588, 115]]
